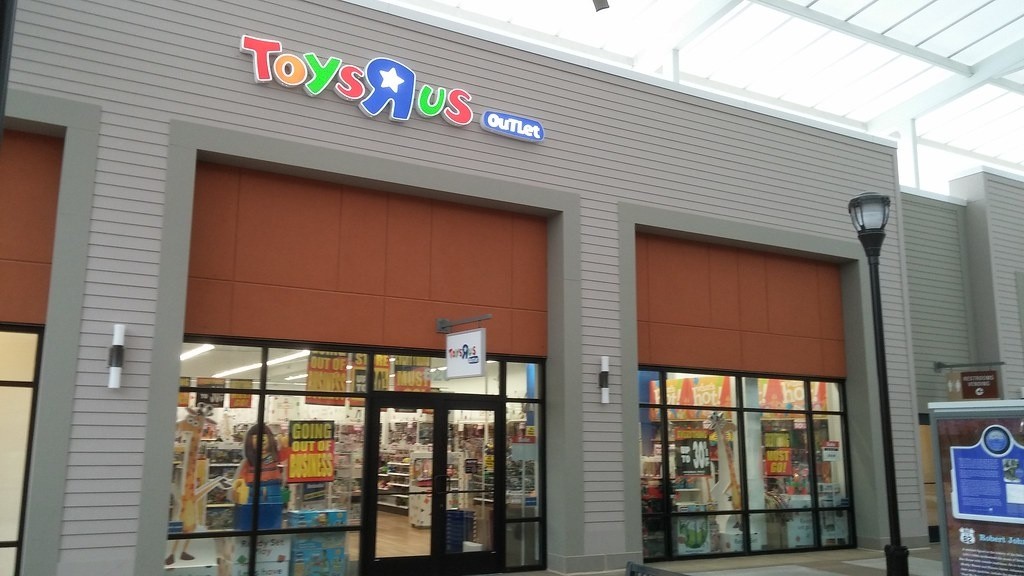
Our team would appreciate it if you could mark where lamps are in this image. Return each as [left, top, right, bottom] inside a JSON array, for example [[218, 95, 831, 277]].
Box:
[[107, 323, 126, 389], [599, 355, 612, 404]]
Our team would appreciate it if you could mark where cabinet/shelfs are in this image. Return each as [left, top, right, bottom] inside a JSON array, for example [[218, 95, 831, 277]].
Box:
[[171, 415, 536, 530], [639, 418, 830, 508]]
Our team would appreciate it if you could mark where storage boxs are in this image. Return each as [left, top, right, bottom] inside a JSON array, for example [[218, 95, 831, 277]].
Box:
[[221, 505, 348, 576], [443, 509, 478, 552], [669, 495, 849, 557]]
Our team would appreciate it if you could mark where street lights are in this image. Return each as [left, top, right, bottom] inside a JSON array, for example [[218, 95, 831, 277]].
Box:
[[846, 189, 917, 575]]
